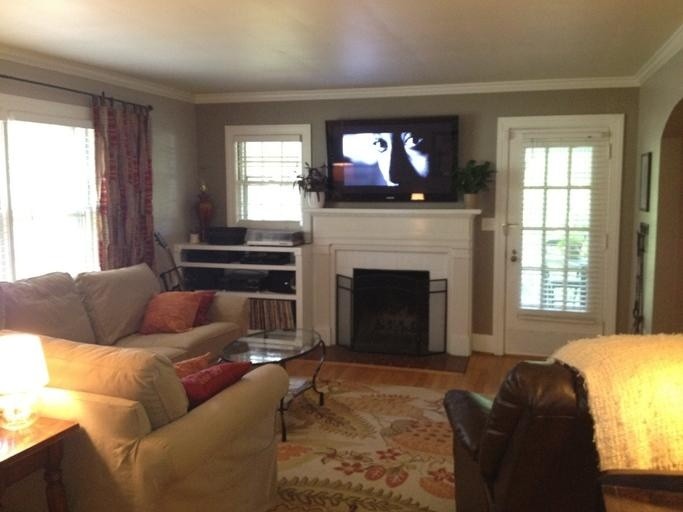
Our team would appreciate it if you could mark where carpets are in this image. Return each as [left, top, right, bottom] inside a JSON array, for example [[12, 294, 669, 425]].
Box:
[[276, 358, 495, 512]]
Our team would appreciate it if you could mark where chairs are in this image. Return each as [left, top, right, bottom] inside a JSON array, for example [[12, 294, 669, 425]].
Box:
[[442, 334, 600, 512]]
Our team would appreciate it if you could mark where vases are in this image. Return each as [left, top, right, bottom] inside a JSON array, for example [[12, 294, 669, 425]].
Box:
[[196, 185, 216, 226]]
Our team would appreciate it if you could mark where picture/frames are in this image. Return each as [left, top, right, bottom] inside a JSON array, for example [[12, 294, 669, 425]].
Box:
[[639, 151, 651, 211]]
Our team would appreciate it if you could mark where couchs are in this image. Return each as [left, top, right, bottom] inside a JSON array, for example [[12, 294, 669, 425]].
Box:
[[0, 330, 290, 512], [0, 263, 251, 362]]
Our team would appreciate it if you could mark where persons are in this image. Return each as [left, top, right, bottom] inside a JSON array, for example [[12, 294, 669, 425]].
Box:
[[373, 130, 434, 187]]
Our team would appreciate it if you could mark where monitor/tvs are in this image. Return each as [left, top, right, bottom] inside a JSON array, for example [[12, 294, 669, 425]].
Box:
[[324, 114, 461, 203]]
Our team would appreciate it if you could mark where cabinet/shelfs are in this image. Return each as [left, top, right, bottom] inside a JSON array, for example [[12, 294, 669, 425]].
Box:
[[174, 244, 314, 352]]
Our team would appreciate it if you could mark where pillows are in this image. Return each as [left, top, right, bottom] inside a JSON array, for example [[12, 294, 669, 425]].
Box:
[[0, 328, 189, 429], [193, 291, 216, 327], [180, 361, 251, 404], [141, 291, 204, 334], [175, 351, 211, 378], [0, 272, 95, 343], [75, 263, 162, 346]]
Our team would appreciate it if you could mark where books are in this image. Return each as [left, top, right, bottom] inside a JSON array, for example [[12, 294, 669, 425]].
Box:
[[250, 298, 295, 330]]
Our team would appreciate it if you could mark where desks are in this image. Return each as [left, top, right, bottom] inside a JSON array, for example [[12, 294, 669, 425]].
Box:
[[0, 416, 80, 512]]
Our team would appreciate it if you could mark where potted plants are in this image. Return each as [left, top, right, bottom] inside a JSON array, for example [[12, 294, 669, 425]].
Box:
[[445, 159, 496, 209], [293, 161, 342, 208]]
